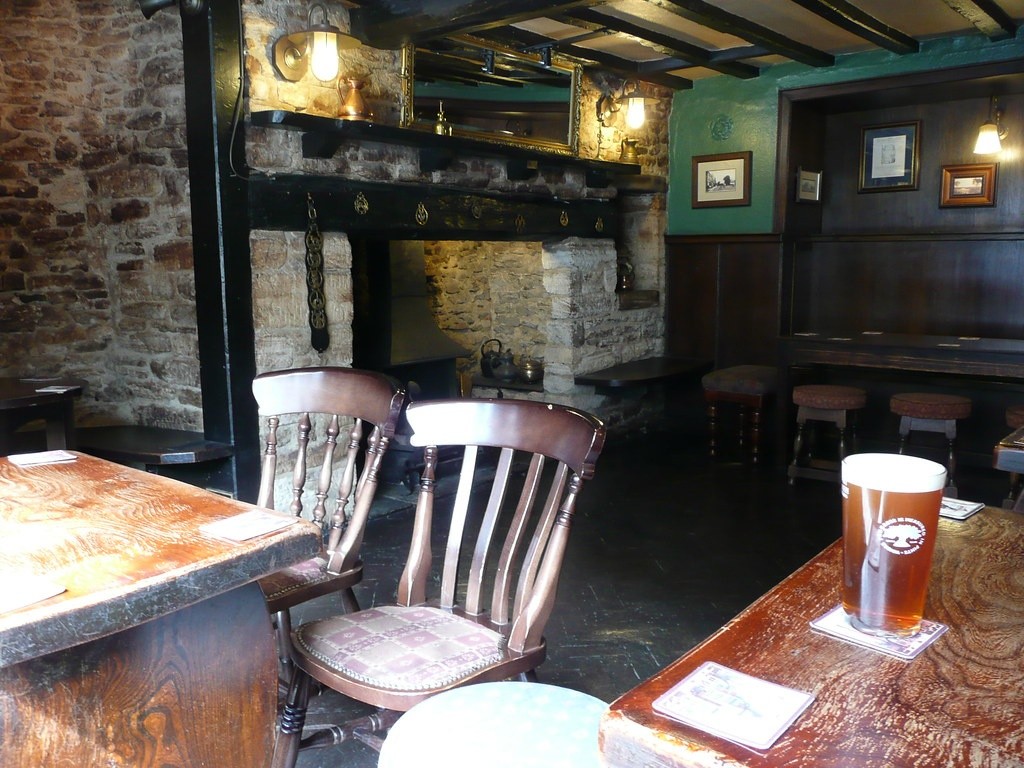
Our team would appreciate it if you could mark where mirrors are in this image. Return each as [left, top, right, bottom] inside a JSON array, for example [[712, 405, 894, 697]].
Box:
[[399, 34, 582, 155]]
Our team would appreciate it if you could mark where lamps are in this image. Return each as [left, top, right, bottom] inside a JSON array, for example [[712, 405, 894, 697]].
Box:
[[540, 47, 552, 68], [973, 92, 1010, 153], [481, 49, 494, 74], [613, 78, 656, 129], [271, 3, 361, 83]]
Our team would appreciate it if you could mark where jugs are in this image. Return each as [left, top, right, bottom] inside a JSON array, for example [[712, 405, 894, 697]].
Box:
[[337, 76, 374, 122], [619, 138, 640, 165]]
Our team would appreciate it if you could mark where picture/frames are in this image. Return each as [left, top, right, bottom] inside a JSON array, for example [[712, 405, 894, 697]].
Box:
[[940, 162, 998, 207], [857, 120, 922, 191], [692, 151, 752, 206], [797, 164, 823, 204]]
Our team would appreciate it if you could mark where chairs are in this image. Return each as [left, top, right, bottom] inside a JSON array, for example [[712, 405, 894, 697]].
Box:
[[250, 367, 406, 703], [272, 396, 609, 768]]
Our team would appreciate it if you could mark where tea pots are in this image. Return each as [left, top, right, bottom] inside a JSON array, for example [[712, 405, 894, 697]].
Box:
[[489, 351, 518, 383], [481, 338, 510, 376]]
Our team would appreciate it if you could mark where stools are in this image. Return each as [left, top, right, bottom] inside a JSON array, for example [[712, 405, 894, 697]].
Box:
[[701, 365, 776, 470], [376, 681, 608, 768], [75, 424, 234, 477], [576, 356, 697, 388], [888, 391, 973, 499], [1001, 404, 1024, 510], [782, 383, 872, 485]]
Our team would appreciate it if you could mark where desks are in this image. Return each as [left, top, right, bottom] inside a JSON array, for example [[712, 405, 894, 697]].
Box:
[[0, 449, 325, 768], [608, 500, 1024, 768], [0, 374, 88, 452]]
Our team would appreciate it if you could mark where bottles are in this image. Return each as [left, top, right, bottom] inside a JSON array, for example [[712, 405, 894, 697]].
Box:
[[433, 112, 452, 136]]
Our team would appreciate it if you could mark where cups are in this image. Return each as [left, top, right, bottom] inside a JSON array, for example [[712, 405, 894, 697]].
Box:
[[840, 451, 948, 638], [520, 359, 542, 384]]
